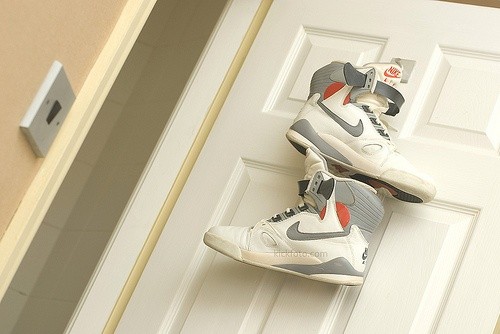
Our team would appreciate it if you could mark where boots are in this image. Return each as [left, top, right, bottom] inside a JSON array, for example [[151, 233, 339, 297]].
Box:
[[285, 57, 440, 205], [203, 147, 386, 286]]
